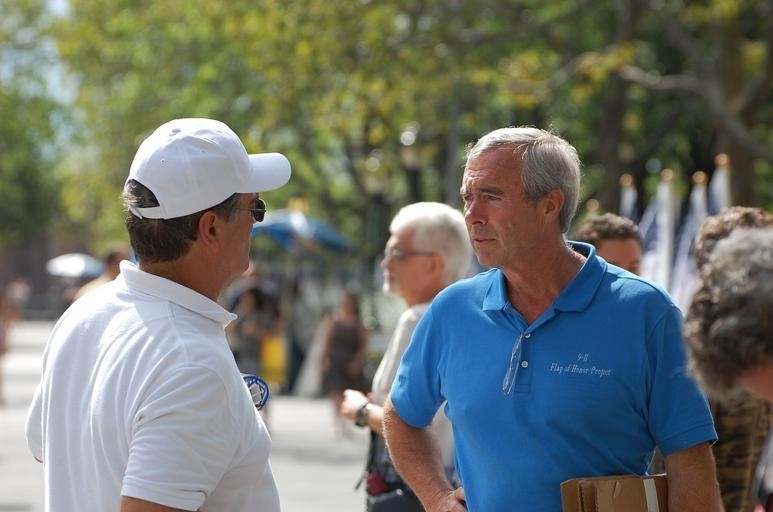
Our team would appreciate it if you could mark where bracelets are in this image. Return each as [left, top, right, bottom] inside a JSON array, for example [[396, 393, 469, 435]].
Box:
[[355, 400, 371, 428]]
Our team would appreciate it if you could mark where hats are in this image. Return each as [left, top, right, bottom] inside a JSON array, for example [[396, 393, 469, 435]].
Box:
[[121, 115, 293, 222]]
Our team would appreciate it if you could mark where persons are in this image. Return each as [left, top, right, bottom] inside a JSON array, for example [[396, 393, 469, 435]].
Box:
[[342, 197, 471, 511], [25, 116, 292, 511], [71, 247, 129, 302], [578, 213, 643, 279], [318, 286, 372, 439], [651, 207, 770, 511], [379, 120, 726, 511]]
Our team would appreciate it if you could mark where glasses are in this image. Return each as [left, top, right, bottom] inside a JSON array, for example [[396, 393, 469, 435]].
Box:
[[213, 199, 270, 224]]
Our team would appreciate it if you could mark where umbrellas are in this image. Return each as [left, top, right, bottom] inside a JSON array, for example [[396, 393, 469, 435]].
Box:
[[45, 251, 106, 279], [251, 208, 356, 256]]
